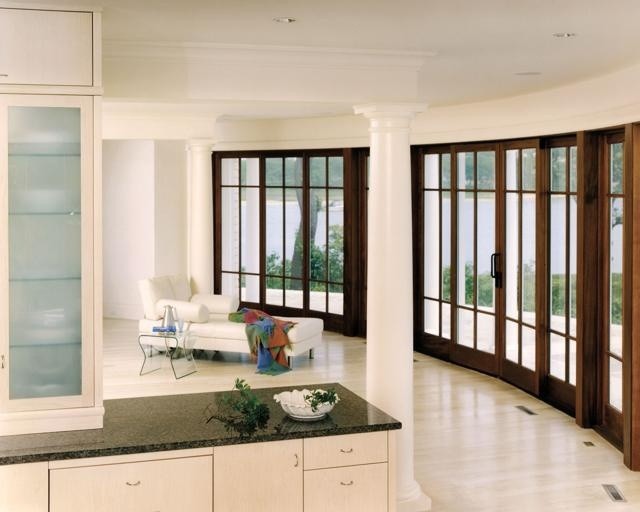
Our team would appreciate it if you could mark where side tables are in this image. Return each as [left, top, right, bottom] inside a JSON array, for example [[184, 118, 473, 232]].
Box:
[[138, 330, 198, 378]]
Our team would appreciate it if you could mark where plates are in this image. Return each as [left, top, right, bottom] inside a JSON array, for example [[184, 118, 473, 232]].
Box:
[[272, 388, 339, 419]]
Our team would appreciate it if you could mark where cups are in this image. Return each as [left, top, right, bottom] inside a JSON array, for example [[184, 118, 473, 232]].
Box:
[[177, 319, 185, 333]]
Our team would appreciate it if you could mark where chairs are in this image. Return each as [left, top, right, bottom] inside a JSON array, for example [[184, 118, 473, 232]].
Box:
[[139, 273, 324, 370]]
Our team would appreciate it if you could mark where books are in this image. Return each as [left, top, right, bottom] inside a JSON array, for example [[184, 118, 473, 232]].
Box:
[[153, 326, 176, 335]]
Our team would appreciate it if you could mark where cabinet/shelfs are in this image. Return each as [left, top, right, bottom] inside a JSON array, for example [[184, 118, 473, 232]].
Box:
[[48, 447, 215, 512], [303, 430, 388, 512], [0, 2, 107, 435], [214, 438, 303, 512]]
